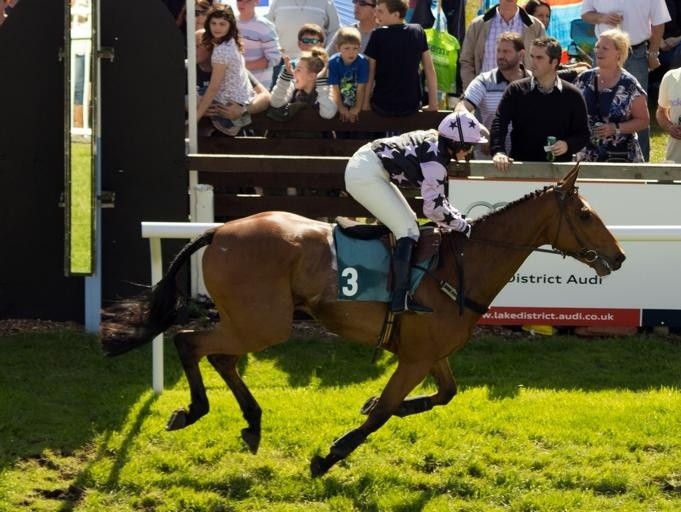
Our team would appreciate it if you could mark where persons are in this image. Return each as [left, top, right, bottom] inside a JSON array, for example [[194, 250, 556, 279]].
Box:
[[343, 111, 481, 313]]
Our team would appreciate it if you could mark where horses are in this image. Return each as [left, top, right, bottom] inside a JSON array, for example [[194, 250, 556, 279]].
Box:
[[98, 161, 626, 478]]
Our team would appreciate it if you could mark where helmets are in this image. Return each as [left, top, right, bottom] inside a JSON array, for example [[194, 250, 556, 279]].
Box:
[[438, 111, 487, 144]]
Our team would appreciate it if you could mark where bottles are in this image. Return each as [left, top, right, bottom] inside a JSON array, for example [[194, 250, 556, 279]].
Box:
[[546, 135, 557, 162], [591, 121, 602, 146]]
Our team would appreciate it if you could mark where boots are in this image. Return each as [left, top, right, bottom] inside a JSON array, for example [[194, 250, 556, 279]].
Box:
[[392, 237, 435, 318]]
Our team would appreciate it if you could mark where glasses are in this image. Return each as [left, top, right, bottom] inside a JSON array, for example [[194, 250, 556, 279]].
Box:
[[301, 38, 319, 43]]
